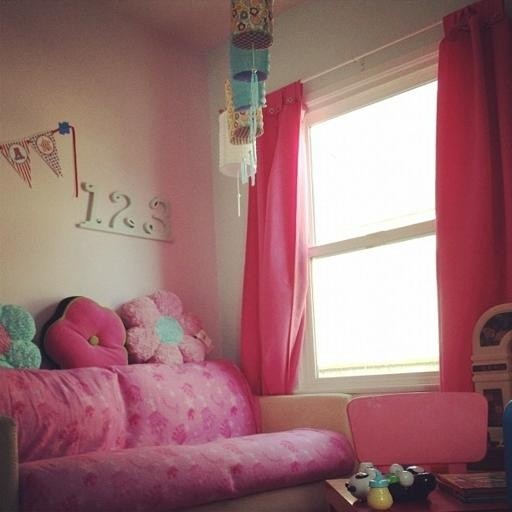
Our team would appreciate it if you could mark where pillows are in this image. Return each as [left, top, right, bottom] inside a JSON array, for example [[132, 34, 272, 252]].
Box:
[[41, 296, 127, 370], [120, 291, 214, 366], [0, 304, 42, 372]]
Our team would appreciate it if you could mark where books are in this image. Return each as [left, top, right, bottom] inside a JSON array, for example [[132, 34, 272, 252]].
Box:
[[433, 466, 510, 503]]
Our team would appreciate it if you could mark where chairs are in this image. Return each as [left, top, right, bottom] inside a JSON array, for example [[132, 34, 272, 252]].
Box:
[[346, 392, 488, 476]]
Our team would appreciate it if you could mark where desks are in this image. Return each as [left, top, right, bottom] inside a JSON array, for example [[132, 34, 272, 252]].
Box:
[[324, 473, 512, 512]]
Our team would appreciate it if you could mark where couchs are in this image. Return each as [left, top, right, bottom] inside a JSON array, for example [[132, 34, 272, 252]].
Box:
[[0, 359, 353, 511]]
